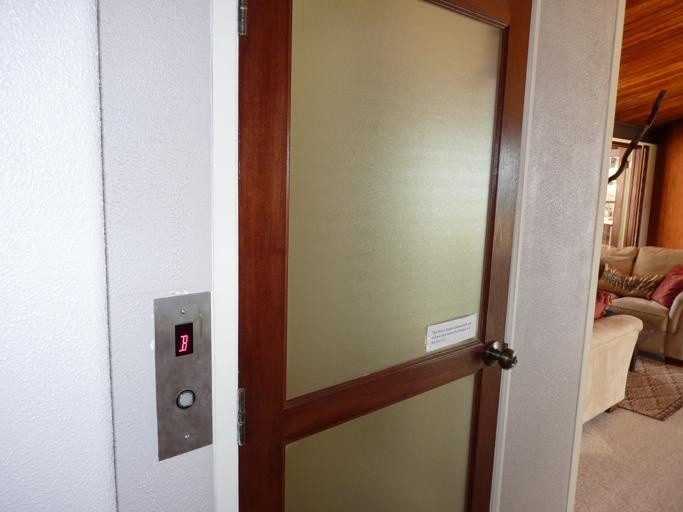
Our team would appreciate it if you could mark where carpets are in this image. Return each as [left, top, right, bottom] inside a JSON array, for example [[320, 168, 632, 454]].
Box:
[[617, 354, 683, 421]]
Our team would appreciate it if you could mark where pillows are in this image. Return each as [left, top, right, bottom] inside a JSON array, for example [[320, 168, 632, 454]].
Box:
[[593, 289, 613, 320], [631, 244, 683, 277], [600, 243, 638, 277], [650, 265, 683, 307], [598, 263, 666, 301]]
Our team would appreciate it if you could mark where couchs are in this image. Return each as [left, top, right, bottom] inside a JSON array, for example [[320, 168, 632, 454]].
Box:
[[581, 313, 644, 424], [596, 242, 683, 364]]
